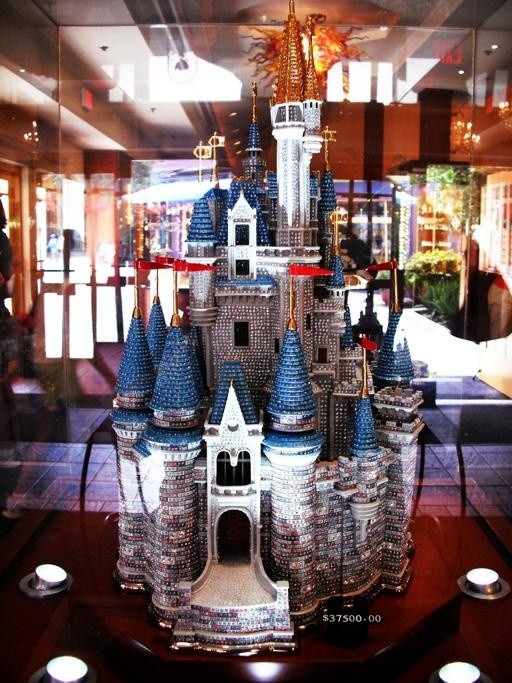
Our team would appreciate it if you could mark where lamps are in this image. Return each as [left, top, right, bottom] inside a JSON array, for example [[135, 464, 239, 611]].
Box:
[[19, 563, 74, 598], [456, 565, 510, 600]]
